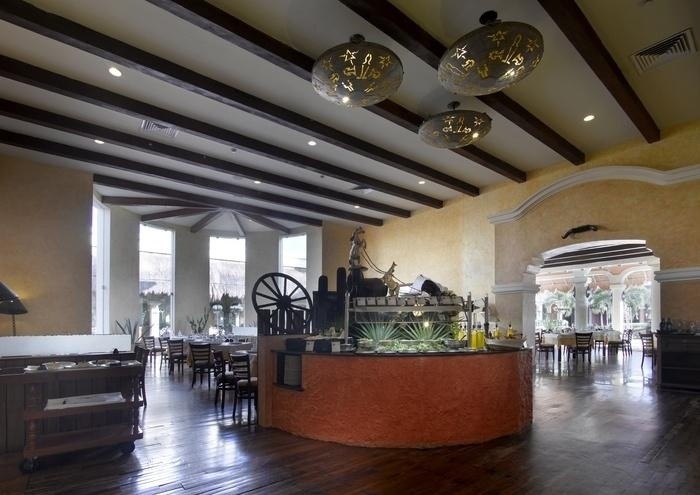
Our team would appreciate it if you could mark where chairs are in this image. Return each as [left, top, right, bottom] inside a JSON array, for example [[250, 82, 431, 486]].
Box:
[[638, 332, 658, 370], [134, 335, 258, 428], [536, 325, 633, 365]]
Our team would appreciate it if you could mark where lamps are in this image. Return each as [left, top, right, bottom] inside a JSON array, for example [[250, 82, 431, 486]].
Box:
[[310, 9, 545, 150]]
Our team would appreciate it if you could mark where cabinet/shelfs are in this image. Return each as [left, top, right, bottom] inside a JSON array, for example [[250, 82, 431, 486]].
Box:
[[18, 359, 145, 462], [655, 330, 700, 395]]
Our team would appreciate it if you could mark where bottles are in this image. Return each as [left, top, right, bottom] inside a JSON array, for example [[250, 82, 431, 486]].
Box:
[[506, 324, 513, 338], [356, 296, 458, 306], [458, 322, 485, 350], [492, 323, 502, 338]]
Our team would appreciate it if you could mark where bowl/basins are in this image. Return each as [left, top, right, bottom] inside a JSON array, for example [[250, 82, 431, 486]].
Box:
[[444, 338, 467, 349], [42, 361, 76, 370]]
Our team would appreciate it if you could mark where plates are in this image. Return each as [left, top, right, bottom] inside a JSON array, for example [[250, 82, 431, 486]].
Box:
[[355, 349, 439, 355]]
